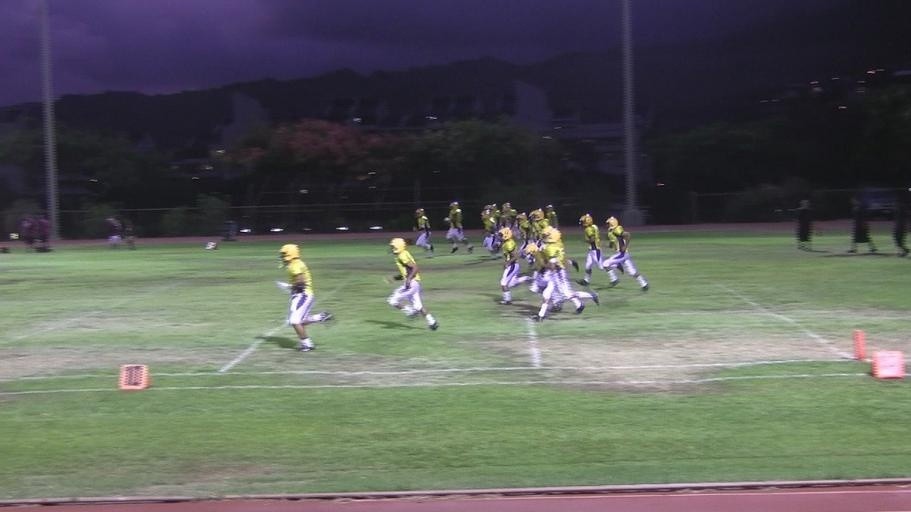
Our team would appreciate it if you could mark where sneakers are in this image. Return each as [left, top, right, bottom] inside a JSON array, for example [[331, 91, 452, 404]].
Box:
[[501, 297, 512, 305], [529, 293, 599, 322], [571, 259, 590, 286], [301, 343, 315, 351], [430, 245, 433, 251], [431, 322, 438, 330], [450, 247, 474, 254], [322, 311, 333, 321], [642, 285, 648, 291], [608, 261, 624, 288], [408, 310, 419, 318]]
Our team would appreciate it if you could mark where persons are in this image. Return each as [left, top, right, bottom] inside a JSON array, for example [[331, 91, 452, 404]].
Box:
[[443, 202, 474, 253], [104, 210, 140, 249], [16, 214, 54, 252], [483, 202, 648, 321], [386, 238, 440, 330], [278, 243, 333, 351], [413, 207, 436, 258], [776, 199, 822, 251], [847, 195, 911, 257]]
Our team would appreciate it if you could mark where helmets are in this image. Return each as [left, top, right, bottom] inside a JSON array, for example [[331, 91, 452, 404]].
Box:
[[450, 202, 458, 210], [481, 203, 561, 243], [416, 209, 424, 217], [389, 237, 406, 254], [278, 243, 300, 262], [606, 216, 618, 226], [579, 213, 593, 227]]
